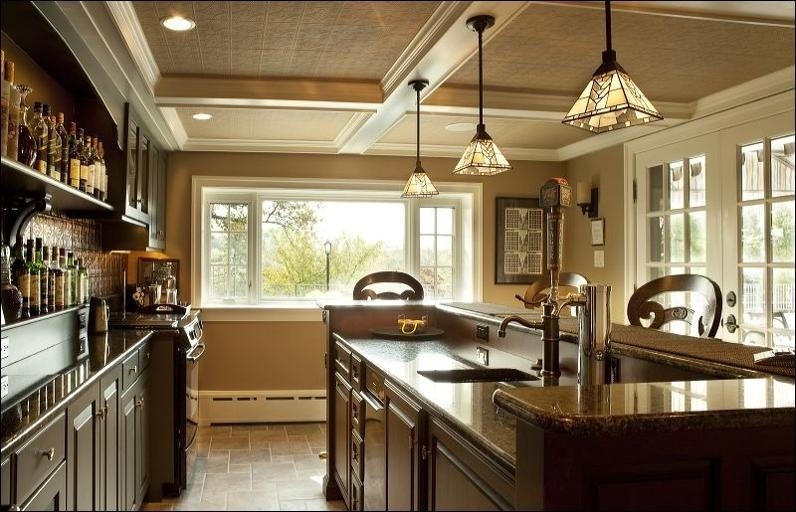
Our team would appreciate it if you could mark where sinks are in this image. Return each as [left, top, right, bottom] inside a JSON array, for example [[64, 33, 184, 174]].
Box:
[[417, 369, 540, 383]]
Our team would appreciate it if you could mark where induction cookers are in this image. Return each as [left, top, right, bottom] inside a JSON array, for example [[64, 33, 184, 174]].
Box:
[[109, 308, 205, 348]]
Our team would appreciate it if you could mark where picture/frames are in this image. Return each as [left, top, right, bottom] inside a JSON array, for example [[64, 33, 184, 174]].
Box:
[[496, 196, 549, 286], [589, 217, 606, 247]]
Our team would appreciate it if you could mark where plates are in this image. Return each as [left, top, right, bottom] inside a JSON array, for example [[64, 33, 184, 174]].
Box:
[[174, 344, 206, 497], [368, 325, 445, 341]]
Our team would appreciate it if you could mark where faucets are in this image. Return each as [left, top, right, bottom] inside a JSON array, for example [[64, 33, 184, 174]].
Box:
[[498, 303, 564, 386]]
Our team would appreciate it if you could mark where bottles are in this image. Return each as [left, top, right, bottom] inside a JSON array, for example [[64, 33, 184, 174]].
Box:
[[1, 60, 22, 162], [74, 256, 90, 306], [57, 111, 70, 186], [31, 236, 46, 316], [28, 99, 49, 176], [59, 246, 72, 307], [83, 134, 97, 194], [41, 245, 56, 314], [11, 236, 33, 321], [67, 252, 81, 307], [0, 244, 24, 324], [98, 137, 111, 202], [50, 245, 66, 311], [76, 127, 94, 192], [43, 101, 63, 182], [92, 134, 105, 201], [29, 239, 42, 316], [68, 118, 83, 190], [16, 81, 38, 168]]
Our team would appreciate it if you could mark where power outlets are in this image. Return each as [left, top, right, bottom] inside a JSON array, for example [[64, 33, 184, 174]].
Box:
[[1, 337, 10, 359], [1, 375, 9, 399]]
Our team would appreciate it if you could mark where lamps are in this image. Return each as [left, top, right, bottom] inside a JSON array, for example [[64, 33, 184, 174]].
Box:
[[400, 79, 440, 197], [577, 181, 598, 218], [560, 1, 665, 134], [449, 14, 513, 178]]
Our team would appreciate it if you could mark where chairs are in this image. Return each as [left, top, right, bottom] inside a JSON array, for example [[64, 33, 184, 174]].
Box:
[[626, 274, 722, 341], [352, 270, 426, 302], [524, 271, 590, 316]]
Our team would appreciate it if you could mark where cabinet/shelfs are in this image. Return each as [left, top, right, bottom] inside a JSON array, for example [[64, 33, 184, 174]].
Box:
[[125, 117, 150, 224], [425, 416, 516, 512], [0, 411, 65, 511], [0, 302, 92, 416], [67, 364, 122, 511], [1, 0, 129, 211], [332, 342, 363, 512], [121, 338, 153, 511], [362, 365, 426, 511], [148, 140, 166, 249], [1, 156, 116, 330]]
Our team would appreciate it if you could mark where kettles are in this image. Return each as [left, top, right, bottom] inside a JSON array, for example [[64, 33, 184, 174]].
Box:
[[89, 296, 110, 334]]
